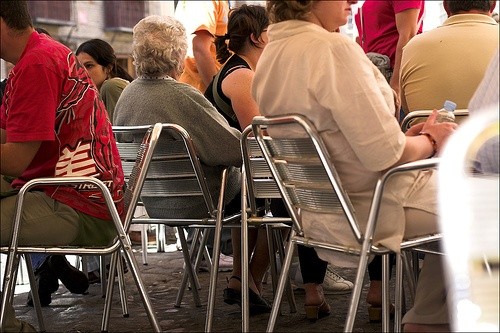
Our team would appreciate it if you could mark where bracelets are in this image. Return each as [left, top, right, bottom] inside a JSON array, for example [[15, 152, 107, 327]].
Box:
[[417, 131, 438, 159]]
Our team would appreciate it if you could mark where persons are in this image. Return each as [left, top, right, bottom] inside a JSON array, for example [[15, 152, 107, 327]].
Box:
[[248, 1, 463, 333], [75, 38, 135, 285], [399, 1, 500, 111], [171, 0, 234, 271], [0, 28, 91, 308], [203, 3, 359, 296], [354, 0, 426, 119], [1, 0, 125, 333], [266, 195, 396, 321], [111, 13, 291, 315]]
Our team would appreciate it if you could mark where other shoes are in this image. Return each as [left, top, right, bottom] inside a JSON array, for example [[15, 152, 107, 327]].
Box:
[[28, 273, 58, 307], [50, 255, 88, 294]]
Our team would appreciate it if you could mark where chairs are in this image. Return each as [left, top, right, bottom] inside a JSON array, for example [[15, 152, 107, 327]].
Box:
[[0, 110, 471, 333]]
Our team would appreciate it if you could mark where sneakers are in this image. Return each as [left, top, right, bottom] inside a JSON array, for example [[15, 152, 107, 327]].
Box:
[[183, 253, 234, 271], [295, 267, 353, 294]]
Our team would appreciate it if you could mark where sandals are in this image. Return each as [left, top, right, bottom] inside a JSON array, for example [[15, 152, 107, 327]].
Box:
[[368, 305, 382, 322], [304, 299, 330, 319], [223, 275, 268, 312]]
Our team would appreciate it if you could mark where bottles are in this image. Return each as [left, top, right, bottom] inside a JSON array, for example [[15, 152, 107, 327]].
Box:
[[434, 98, 458, 124]]
